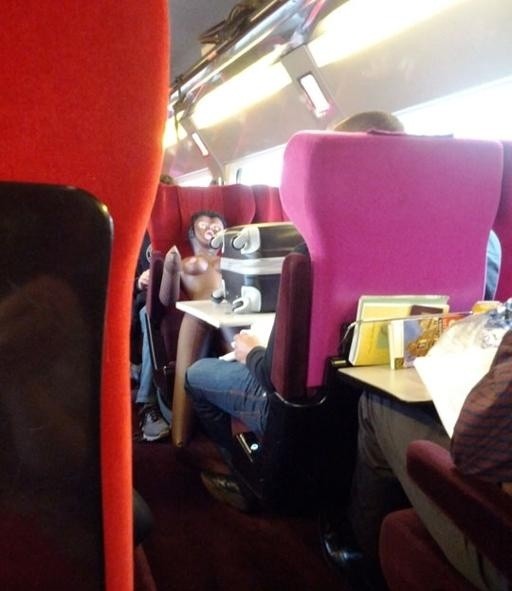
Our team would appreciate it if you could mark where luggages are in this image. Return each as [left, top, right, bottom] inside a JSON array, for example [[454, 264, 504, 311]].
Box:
[[210, 221, 304, 314]]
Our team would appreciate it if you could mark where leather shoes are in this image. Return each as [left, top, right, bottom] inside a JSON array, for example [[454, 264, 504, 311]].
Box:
[[321, 535, 365, 572]]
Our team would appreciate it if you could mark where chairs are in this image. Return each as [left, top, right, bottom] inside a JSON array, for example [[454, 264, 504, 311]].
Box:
[[4, 1, 512, 591]]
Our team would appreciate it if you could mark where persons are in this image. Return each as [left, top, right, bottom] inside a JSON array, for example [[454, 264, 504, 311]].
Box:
[[183, 112, 405, 515], [125, 175, 176, 445], [159, 212, 228, 448], [314, 331, 510, 591]]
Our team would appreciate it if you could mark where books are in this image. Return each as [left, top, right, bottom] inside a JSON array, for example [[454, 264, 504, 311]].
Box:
[[348, 294, 474, 371]]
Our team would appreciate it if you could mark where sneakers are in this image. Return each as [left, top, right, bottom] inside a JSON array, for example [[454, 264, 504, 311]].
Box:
[[200, 469, 256, 514], [139, 408, 170, 442]]
